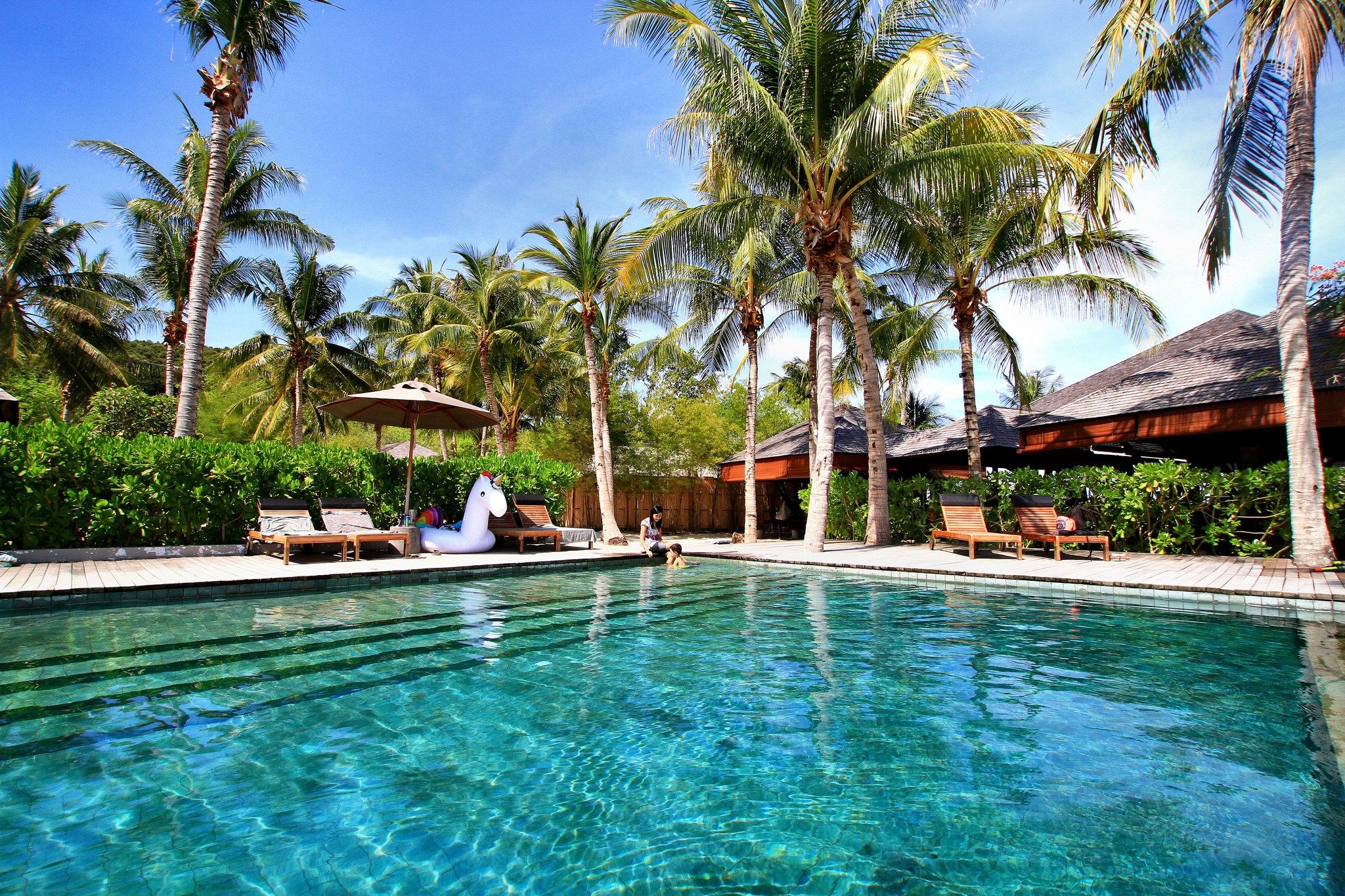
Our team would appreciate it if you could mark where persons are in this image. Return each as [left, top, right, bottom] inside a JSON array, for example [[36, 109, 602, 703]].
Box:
[[669, 543, 686, 566], [640, 505, 687, 563]]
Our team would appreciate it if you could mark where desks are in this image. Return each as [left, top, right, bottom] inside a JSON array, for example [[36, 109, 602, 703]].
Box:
[[389, 526, 420, 554]]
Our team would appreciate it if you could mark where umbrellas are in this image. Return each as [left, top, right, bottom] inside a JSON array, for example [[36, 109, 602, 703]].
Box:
[[316, 377, 501, 515]]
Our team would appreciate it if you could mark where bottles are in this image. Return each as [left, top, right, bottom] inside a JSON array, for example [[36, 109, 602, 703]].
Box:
[[398, 513, 410, 527]]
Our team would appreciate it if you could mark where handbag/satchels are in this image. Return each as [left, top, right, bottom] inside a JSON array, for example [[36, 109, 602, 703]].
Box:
[[1055, 516, 1076, 535]]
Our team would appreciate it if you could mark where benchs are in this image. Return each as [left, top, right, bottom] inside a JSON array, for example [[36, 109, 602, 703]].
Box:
[[318, 498, 410, 560], [248, 497, 347, 565], [930, 493, 1022, 560], [488, 493, 597, 553], [999, 494, 1110, 561]]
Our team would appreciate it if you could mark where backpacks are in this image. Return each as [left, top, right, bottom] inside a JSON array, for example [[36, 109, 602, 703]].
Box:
[[1066, 504, 1098, 536]]
